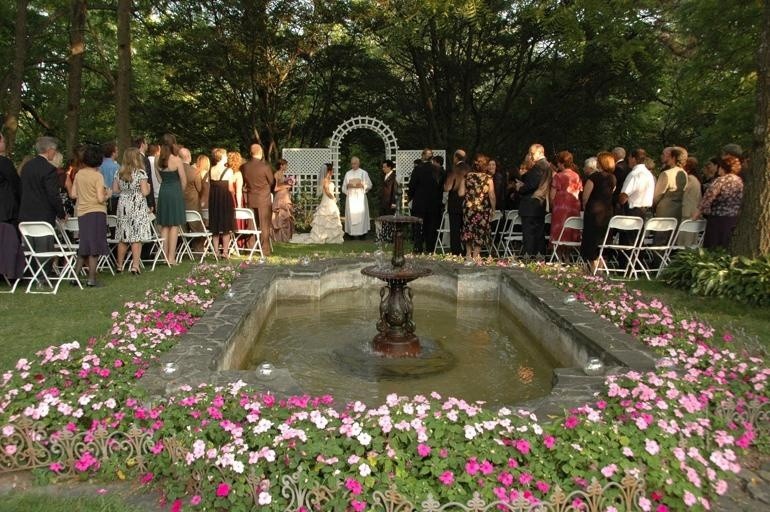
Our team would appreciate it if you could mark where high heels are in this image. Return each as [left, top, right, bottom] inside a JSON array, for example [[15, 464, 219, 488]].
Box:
[[131, 267, 142, 275], [116, 267, 123, 273]]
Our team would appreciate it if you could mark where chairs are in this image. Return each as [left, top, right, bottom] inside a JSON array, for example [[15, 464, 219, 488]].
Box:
[[0, 206, 266, 296], [433, 209, 710, 282]]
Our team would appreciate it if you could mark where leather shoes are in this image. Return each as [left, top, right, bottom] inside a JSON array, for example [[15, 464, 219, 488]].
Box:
[[71, 280, 83, 285], [87, 278, 96, 286]]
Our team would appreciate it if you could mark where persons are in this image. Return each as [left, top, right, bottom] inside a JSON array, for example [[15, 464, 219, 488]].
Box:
[[289, 163, 345, 244], [406, 144, 743, 277], [379, 159, 398, 243], [342, 156, 373, 240], [0, 131, 297, 287]]
